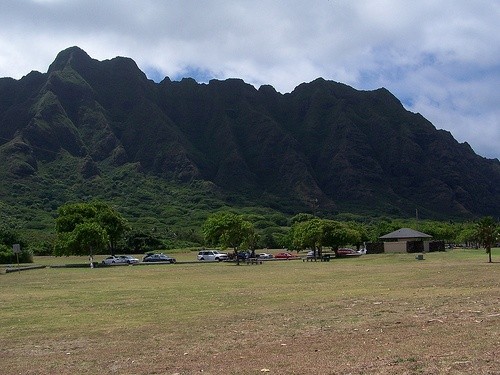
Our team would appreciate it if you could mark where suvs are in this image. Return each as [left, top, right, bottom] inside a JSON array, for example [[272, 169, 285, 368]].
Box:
[[197, 250, 228, 261]]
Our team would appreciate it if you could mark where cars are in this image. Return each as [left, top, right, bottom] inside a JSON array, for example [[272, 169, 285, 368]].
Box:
[[102, 256, 123, 265], [143, 253, 176, 263], [307, 250, 321, 258], [274, 252, 292, 258], [253, 253, 271, 260], [120, 256, 139, 264], [235, 249, 252, 259], [337, 248, 358, 256]]
